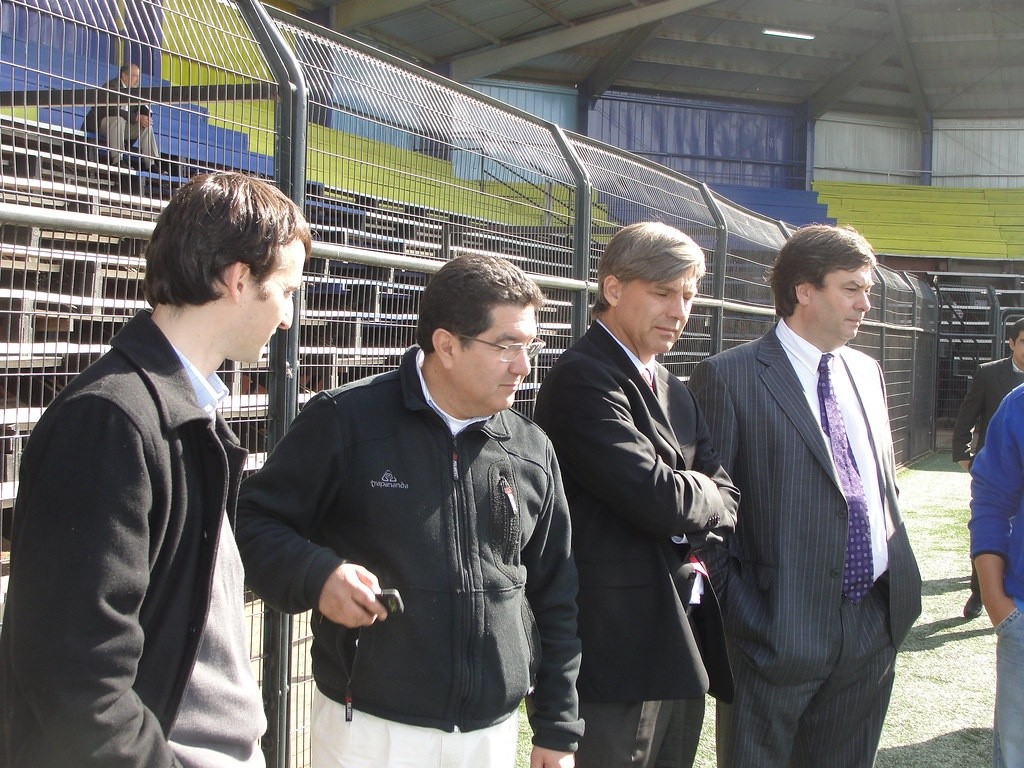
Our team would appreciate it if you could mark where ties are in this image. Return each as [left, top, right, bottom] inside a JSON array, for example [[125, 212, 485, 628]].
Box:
[[817, 352, 874, 606], [641, 368, 710, 579]]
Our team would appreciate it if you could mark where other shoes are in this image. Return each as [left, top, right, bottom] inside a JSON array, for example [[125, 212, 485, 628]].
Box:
[[962, 581, 983, 620], [113, 158, 135, 171], [144, 164, 169, 175]]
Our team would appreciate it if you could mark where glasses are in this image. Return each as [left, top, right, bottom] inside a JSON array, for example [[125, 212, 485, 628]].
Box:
[[450, 331, 546, 363]]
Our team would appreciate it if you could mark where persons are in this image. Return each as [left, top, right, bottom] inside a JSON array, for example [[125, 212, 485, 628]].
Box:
[[688, 222, 925, 768], [234, 252, 586, 768], [951, 317, 1024, 767], [80, 64, 168, 175], [533, 218, 743, 768], [0, 171, 314, 768]]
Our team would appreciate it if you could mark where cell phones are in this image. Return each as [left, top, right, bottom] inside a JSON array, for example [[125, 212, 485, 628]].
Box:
[[375, 589, 404, 615]]
